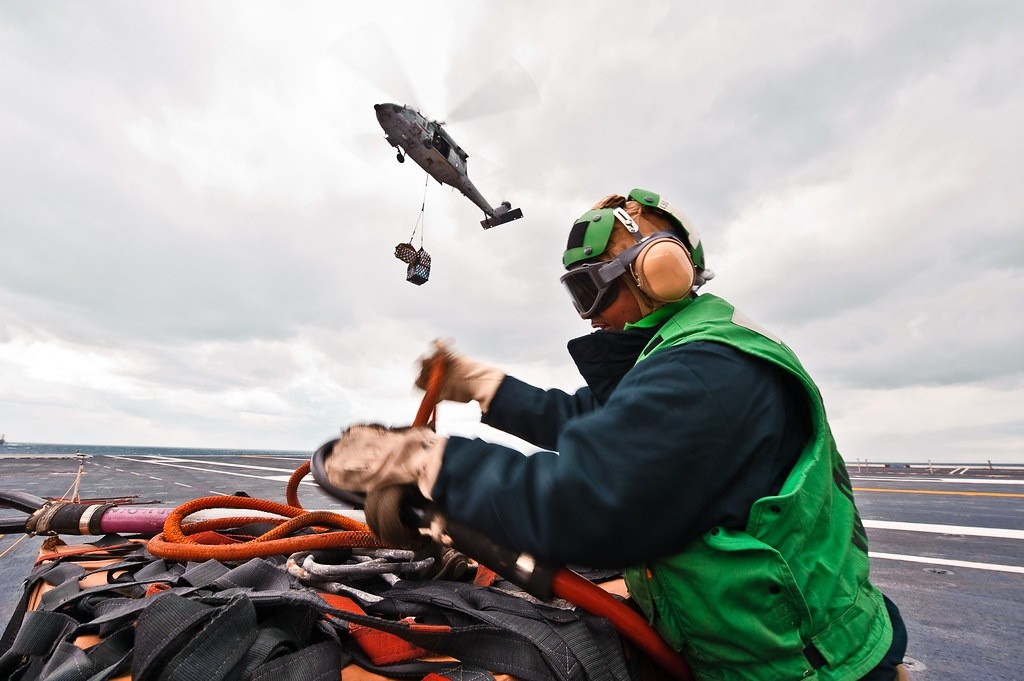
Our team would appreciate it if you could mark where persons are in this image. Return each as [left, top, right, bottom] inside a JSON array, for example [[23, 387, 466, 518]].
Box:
[[324, 188, 907, 681], [434, 135, 442, 151]]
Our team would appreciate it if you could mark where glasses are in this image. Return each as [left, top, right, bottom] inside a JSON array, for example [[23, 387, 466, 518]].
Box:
[[560, 233, 674, 319]]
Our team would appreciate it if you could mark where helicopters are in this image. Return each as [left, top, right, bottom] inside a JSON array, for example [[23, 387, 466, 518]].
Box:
[[337, 22, 542, 230]]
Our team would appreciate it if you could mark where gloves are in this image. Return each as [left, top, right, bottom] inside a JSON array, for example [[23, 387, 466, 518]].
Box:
[[414, 338, 505, 413], [324, 425, 447, 500]]
